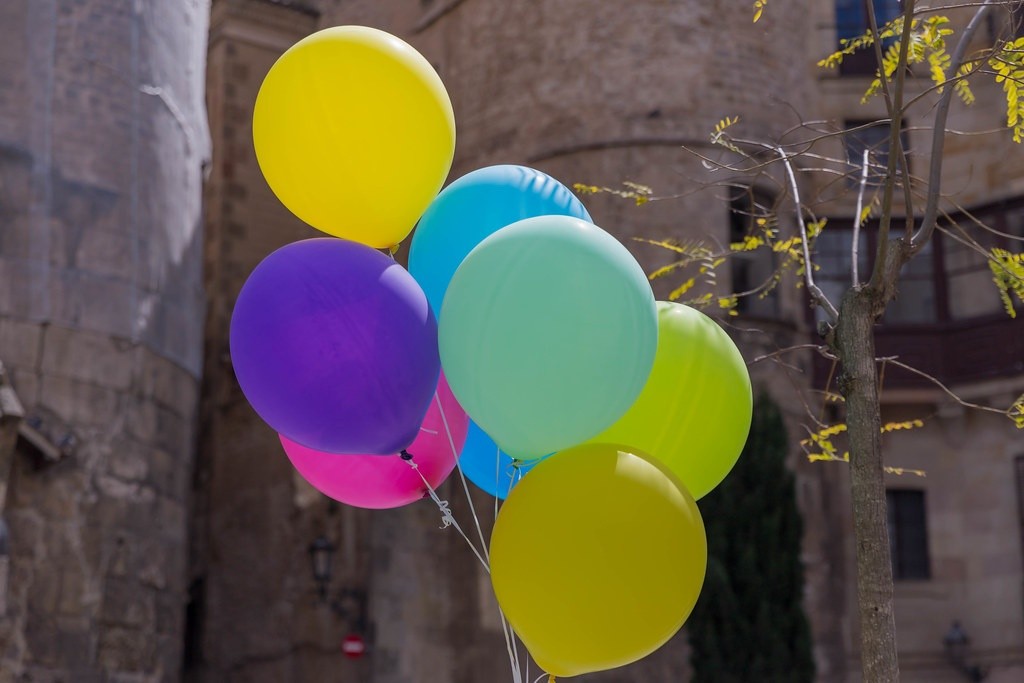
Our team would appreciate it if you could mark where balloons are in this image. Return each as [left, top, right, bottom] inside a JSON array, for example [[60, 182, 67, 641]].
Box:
[[438, 215, 659, 471], [559, 301, 753, 502], [277, 367, 469, 510], [253, 24, 456, 255], [230, 237, 441, 460], [457, 418, 559, 501], [489, 444, 707, 683], [408, 165, 595, 323]]
